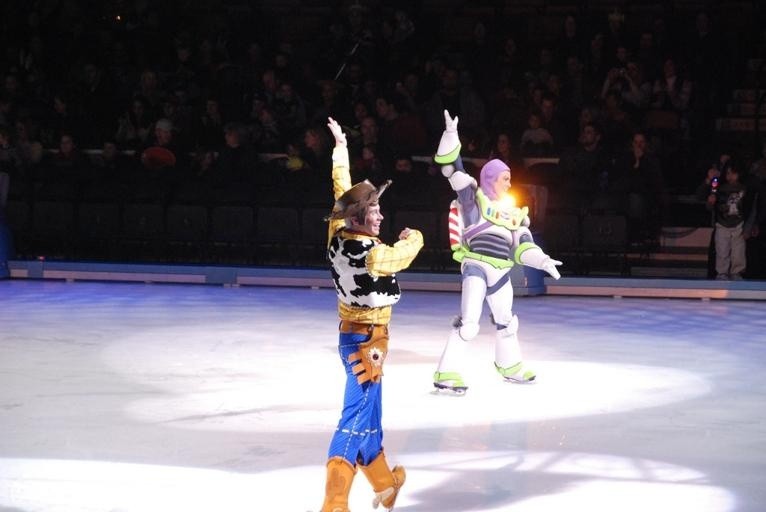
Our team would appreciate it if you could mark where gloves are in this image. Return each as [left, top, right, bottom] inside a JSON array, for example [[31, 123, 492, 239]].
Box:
[[541, 255, 565, 281], [443, 107, 461, 134]]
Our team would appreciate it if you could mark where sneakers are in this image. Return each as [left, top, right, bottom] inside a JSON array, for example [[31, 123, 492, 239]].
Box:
[[491, 358, 538, 382], [431, 368, 471, 393]]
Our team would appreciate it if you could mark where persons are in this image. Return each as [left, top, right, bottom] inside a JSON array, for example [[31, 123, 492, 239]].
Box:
[[426, 101, 564, 394], [316, 112, 429, 512], [0, 0, 766, 283]]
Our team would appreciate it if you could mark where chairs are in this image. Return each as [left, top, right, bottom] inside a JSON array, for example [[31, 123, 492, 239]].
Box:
[[0, 0, 766, 270]]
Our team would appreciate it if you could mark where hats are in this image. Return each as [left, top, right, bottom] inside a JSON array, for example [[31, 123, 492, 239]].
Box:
[[317, 177, 395, 224]]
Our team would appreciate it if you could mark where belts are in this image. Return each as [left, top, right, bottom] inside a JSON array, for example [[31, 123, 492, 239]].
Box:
[[335, 319, 390, 337]]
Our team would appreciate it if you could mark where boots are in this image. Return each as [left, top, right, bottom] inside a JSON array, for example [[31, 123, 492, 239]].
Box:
[[354, 444, 409, 512], [318, 457, 358, 512]]
